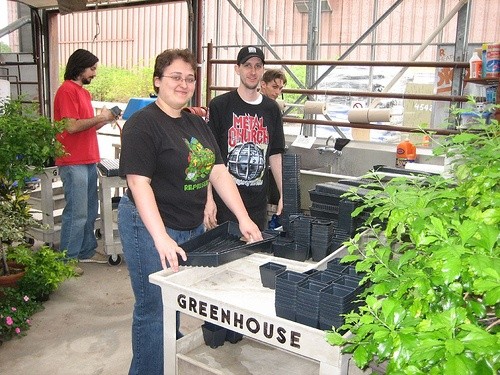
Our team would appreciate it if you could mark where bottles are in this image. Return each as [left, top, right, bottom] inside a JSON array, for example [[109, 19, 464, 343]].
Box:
[[469, 52, 482, 78]]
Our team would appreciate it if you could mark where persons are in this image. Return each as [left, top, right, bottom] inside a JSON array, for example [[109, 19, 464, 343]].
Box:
[[203, 45, 285, 233], [54, 49, 123, 263], [260, 70, 287, 101], [117, 49, 264, 375]]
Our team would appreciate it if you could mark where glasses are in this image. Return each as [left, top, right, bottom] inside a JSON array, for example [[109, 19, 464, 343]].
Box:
[[160, 75, 196, 84]]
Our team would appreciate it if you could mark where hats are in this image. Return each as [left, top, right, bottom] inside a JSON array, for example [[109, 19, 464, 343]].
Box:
[[236, 45, 264, 67]]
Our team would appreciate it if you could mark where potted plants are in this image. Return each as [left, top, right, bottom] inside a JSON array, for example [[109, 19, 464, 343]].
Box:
[[0, 94, 79, 345]]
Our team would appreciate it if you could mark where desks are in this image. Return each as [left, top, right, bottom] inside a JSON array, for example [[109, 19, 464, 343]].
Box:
[[146, 251, 371, 375]]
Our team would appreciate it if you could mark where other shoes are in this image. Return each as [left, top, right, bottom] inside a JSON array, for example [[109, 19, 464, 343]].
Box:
[[72, 266, 84, 277], [78, 252, 111, 264]]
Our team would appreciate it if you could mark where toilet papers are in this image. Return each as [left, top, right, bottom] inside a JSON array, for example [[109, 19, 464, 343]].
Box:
[[368, 110, 392, 123], [304, 101, 327, 114], [349, 108, 368, 124], [274, 102, 289, 111]]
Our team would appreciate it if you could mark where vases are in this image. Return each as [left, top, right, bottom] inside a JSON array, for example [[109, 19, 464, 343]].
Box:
[[168, 150, 440, 338]]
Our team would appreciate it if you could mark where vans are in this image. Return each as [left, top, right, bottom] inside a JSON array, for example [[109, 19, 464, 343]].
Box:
[[314, 75, 392, 112]]
